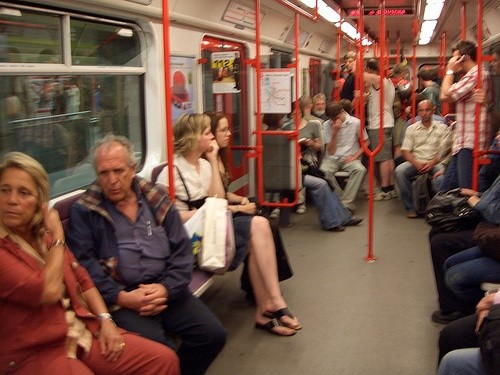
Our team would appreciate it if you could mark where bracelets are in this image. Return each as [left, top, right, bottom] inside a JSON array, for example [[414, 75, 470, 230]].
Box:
[[47, 239, 66, 250], [96, 313, 111, 321]]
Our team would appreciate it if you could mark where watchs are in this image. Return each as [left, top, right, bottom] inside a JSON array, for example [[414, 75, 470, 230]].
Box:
[[487, 102, 495, 112], [445, 69, 454, 75]]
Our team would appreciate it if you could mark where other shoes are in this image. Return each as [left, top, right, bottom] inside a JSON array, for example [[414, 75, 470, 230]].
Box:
[[297, 203, 307, 213], [390, 189, 398, 197], [270, 209, 280, 218], [344, 218, 363, 226], [408, 209, 418, 217], [432, 310, 456, 323], [330, 225, 345, 230], [374, 191, 391, 200]]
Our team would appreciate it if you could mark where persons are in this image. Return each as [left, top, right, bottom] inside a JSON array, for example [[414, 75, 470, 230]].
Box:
[[154, 113, 303, 335], [264, 39, 500, 375], [68, 133, 230, 375], [203, 110, 295, 298], [0, 152, 181, 375]]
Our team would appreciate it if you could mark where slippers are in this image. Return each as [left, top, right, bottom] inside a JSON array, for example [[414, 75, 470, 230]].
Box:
[[255, 318, 296, 336], [263, 306, 303, 331]]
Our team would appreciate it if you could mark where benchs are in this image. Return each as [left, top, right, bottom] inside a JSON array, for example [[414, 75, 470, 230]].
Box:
[[46, 183, 221, 299]]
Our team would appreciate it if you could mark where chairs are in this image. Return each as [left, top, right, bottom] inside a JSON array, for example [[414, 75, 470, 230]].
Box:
[[332, 151, 363, 177]]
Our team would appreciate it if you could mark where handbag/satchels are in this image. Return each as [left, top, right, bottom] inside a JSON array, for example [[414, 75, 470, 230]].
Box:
[[426, 188, 481, 232], [182, 196, 237, 273]]
